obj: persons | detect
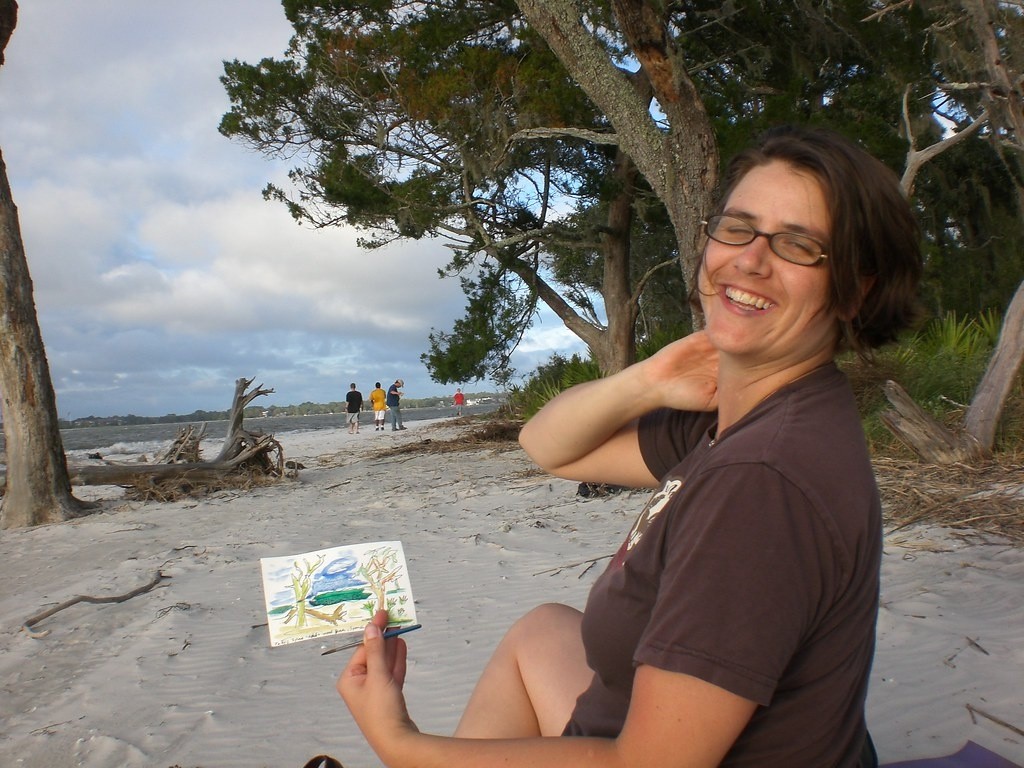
[368,382,387,431]
[345,383,364,434]
[337,129,935,768]
[387,379,408,431]
[454,387,464,417]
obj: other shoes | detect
[392,428,397,431]
[399,427,408,430]
[349,431,354,434]
[381,427,384,430]
[375,428,379,431]
[355,432,359,433]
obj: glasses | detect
[701,214,830,267]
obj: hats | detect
[397,379,404,388]
[457,388,461,391]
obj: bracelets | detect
[346,407,348,409]
